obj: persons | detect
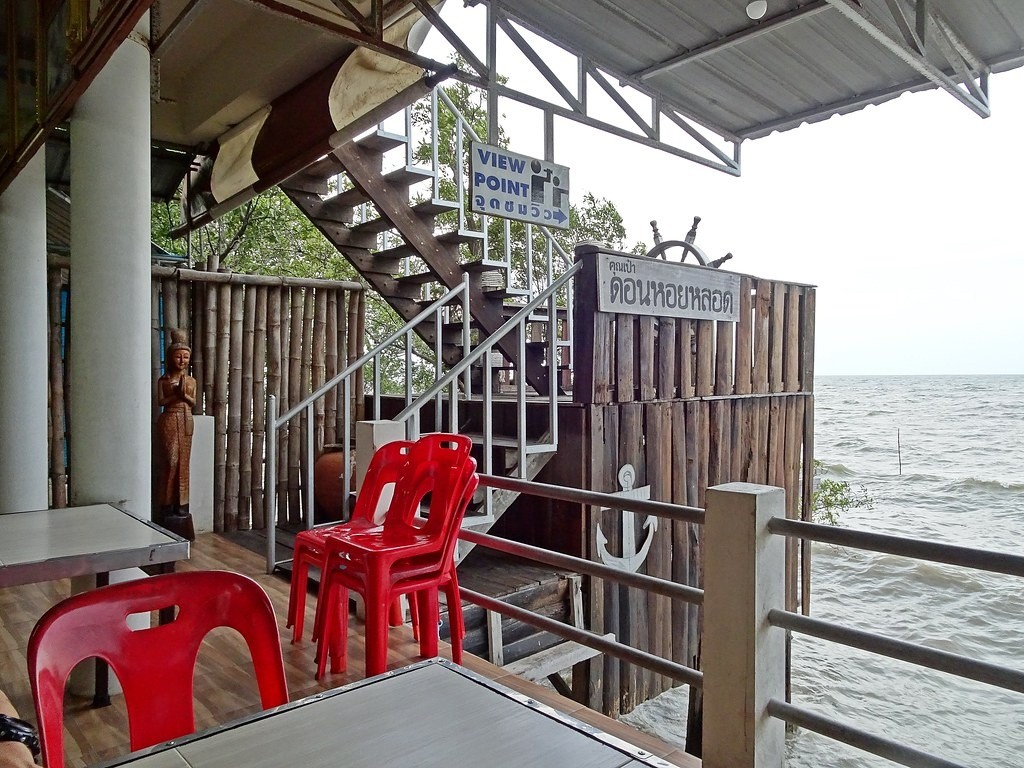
[0,689,44,768]
[158,329,197,516]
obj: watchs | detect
[0,713,41,764]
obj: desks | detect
[86,656,703,768]
[0,501,191,708]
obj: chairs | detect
[286,433,479,680]
[26,570,289,768]
